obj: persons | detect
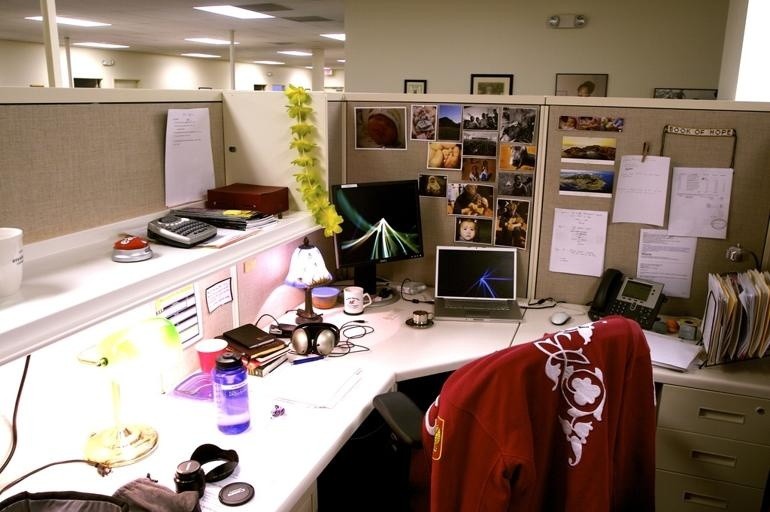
[561,115,624,131]
[577,81,595,97]
[356,103,535,247]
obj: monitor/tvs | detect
[330,178,425,309]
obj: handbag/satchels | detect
[110,478,199,512]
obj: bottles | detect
[212,350,252,435]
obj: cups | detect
[195,337,229,373]
[412,309,433,323]
[0,228,23,297]
[343,285,373,316]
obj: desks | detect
[0,87,770,511]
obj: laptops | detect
[434,244,523,323]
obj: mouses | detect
[551,311,572,325]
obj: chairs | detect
[373,315,660,512]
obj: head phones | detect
[291,322,340,357]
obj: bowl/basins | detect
[311,286,340,309]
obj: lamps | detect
[286,238,332,325]
[548,13,586,29]
[75,318,182,467]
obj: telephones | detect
[590,269,665,329]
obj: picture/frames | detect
[654,87,718,100]
[555,73,608,96]
[404,79,427,94]
[471,73,514,95]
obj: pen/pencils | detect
[292,355,323,363]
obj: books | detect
[213,322,291,378]
[170,207,279,232]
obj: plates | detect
[407,319,435,329]
